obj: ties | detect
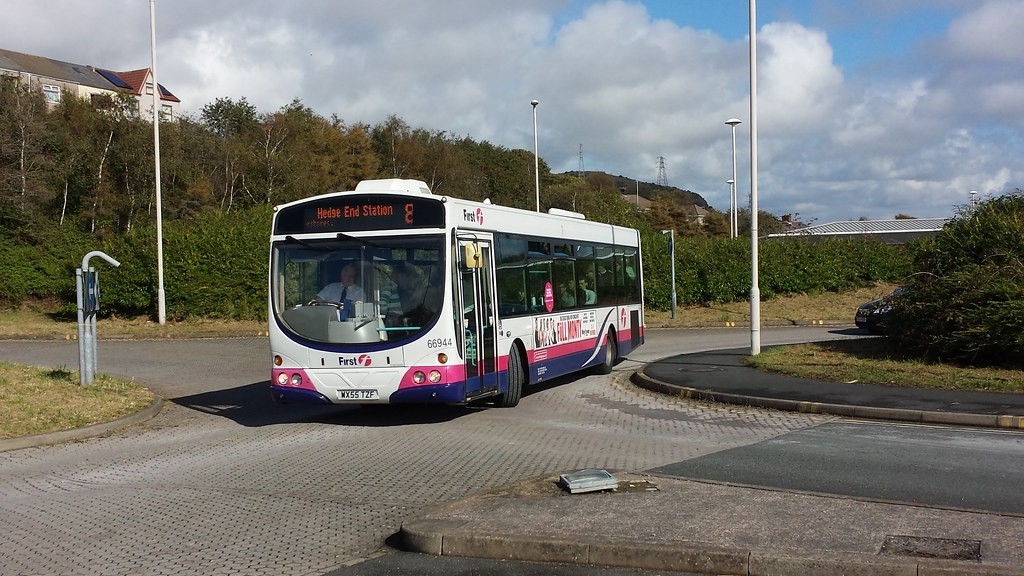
[339,286,348,306]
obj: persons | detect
[463,273,477,338]
[380,265,410,334]
[309,265,367,321]
[585,260,635,287]
[535,318,557,348]
[556,277,595,307]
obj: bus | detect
[263,178,646,415]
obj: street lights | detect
[723,118,746,237]
[531,100,543,211]
[725,179,736,240]
[661,228,681,320]
[970,190,979,217]
